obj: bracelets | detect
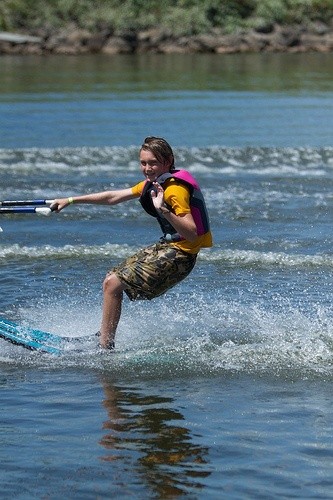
[68,197,74,205]
[158,206,171,216]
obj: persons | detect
[50,136,212,351]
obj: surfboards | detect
[1,318,98,357]
